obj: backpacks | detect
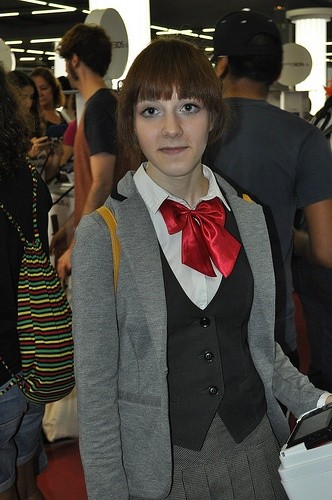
[17,241,75,405]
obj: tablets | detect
[46,124,68,141]
[287,402,332,448]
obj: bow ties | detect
[159,197,242,278]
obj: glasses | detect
[212,54,229,63]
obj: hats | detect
[209,12,283,55]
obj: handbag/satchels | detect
[42,385,82,441]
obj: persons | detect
[0,11,332,500]
[71,38,332,500]
